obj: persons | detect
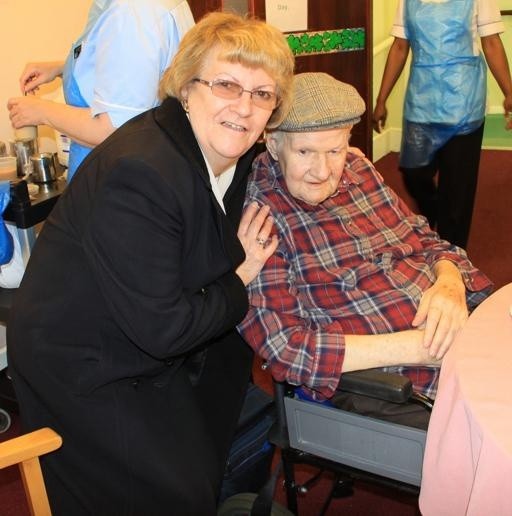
[221,70,503,430]
[367,0,512,257]
[4,9,301,516]
[6,0,186,185]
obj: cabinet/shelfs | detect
[0,177,71,410]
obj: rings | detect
[256,235,266,246]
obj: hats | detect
[265,71,366,133]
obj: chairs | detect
[0,406,64,516]
[248,355,436,515]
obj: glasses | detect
[193,77,283,110]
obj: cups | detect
[0,122,61,183]
